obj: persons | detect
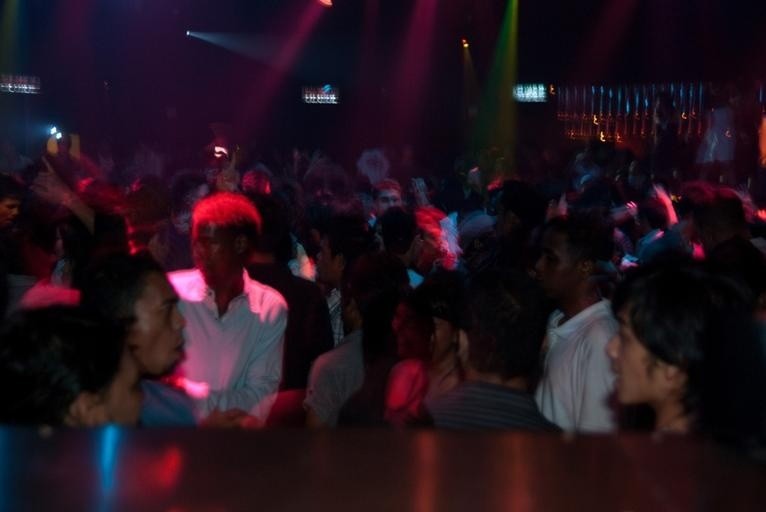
[2,85,765,434]
